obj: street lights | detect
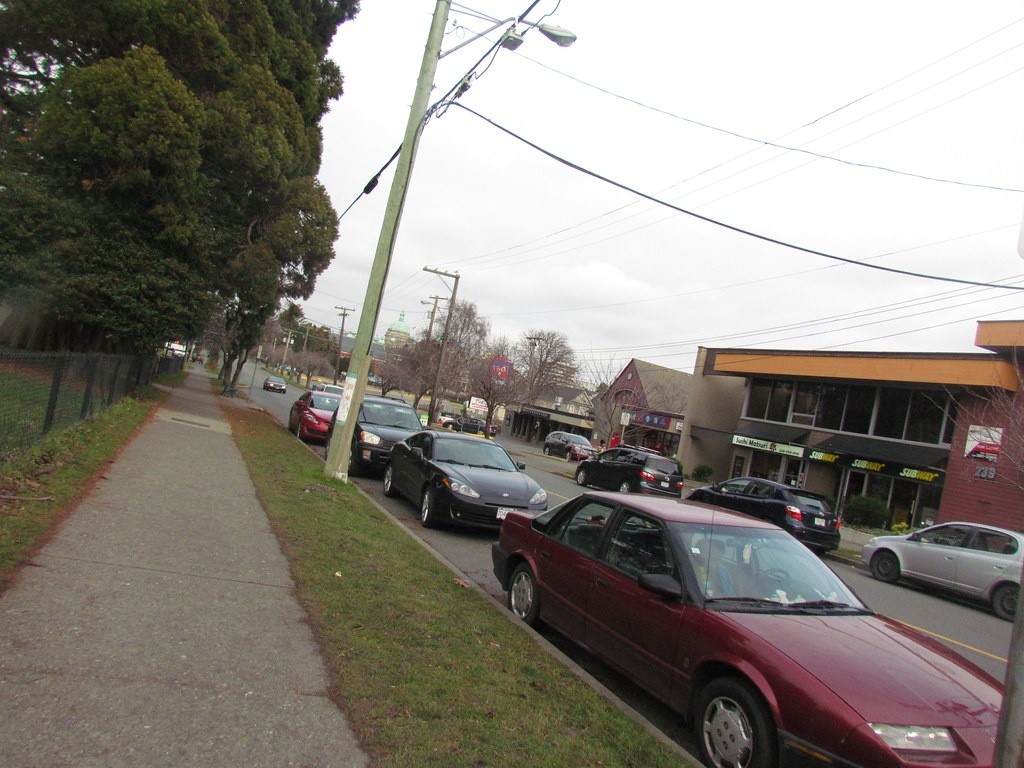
[279,337,294,367]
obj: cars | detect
[443,417,496,438]
[263,376,287,393]
[384,430,547,535]
[493,489,1006,768]
[279,365,297,371]
[861,521,1024,624]
[320,384,344,395]
[289,391,342,443]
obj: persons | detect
[597,440,606,453]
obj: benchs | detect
[567,526,662,566]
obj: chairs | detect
[696,539,732,591]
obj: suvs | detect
[685,475,842,555]
[574,444,684,500]
[542,431,593,462]
[324,394,427,477]
